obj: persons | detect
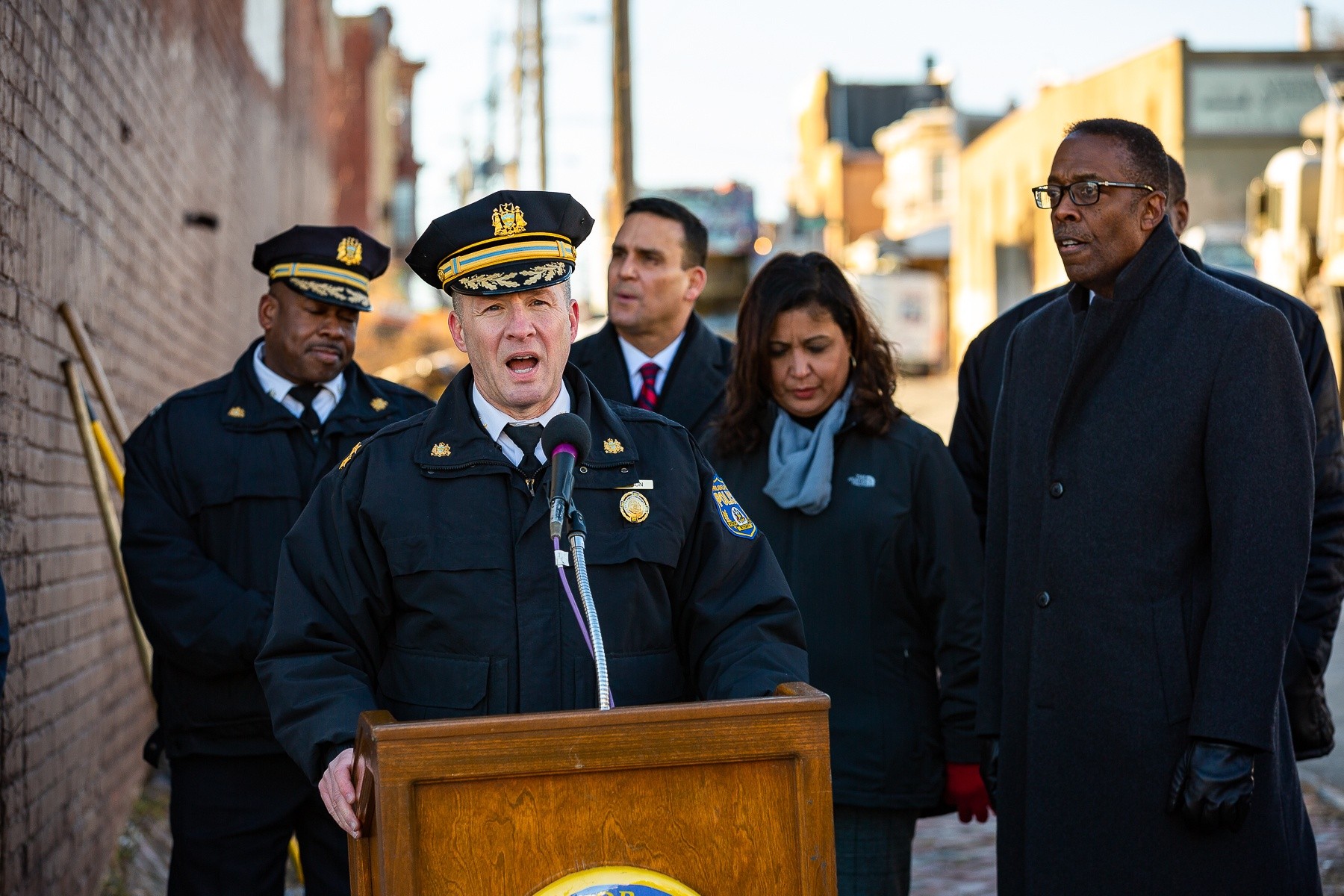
[254,190,810,841]
[1160,156,1344,763]
[119,224,437,896]
[977,118,1324,896]
[694,251,993,896]
[564,199,744,444]
[946,279,1075,798]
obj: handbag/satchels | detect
[908,809,997,896]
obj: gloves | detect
[943,760,995,824]
[1170,742,1254,823]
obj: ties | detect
[505,422,544,478]
[290,385,322,440]
[635,361,661,413]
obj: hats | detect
[404,189,601,295]
[251,223,393,312]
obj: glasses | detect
[1032,182,1155,209]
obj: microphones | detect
[542,413,591,539]
